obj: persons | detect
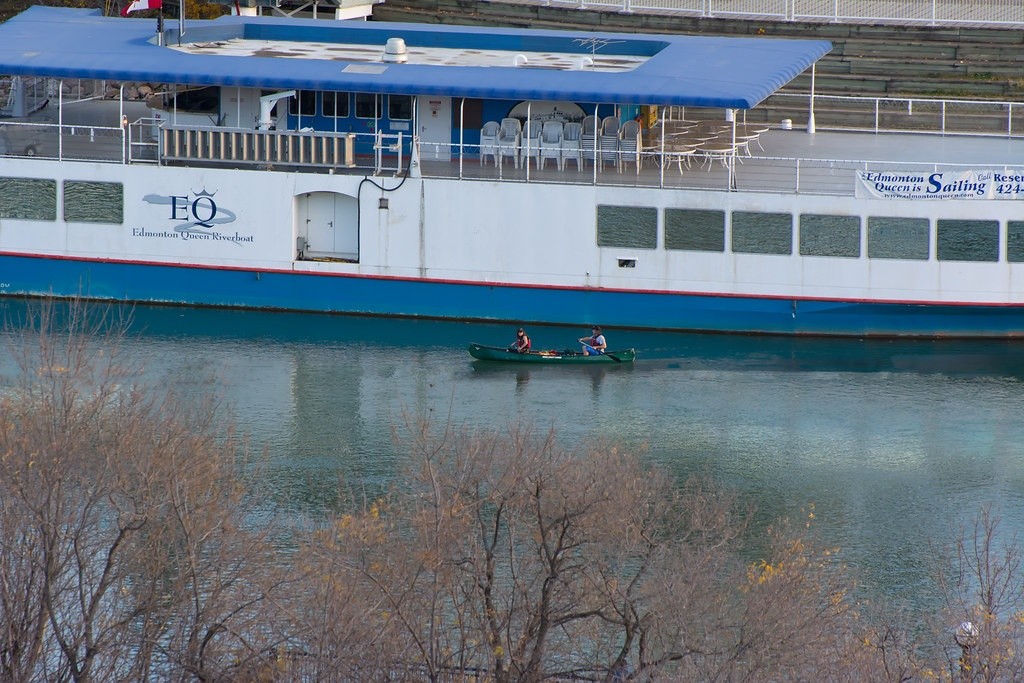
[578,326,606,356]
[506,328,531,354]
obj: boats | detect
[0,0,1022,336]
[465,339,636,364]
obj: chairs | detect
[498,118,521,169]
[521,120,542,169]
[579,115,601,174]
[562,122,581,172]
[540,121,563,171]
[619,120,642,176]
[599,116,619,175]
[479,121,499,167]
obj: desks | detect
[642,118,769,170]
[695,143,737,173]
[654,144,697,174]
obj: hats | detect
[516,327,524,331]
[592,326,601,330]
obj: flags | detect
[120,0,162,16]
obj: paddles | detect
[579,341,621,363]
